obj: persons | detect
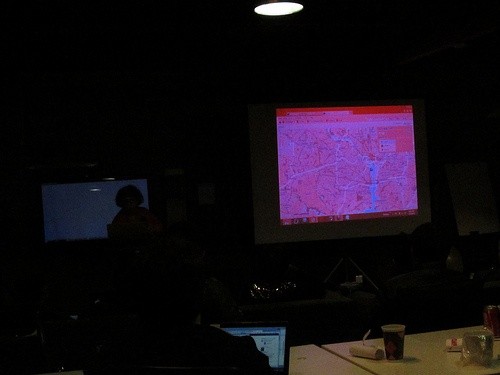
[127,269,275,375]
[106,186,158,237]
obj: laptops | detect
[220,321,289,375]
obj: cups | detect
[381,323,406,360]
[460,326,494,367]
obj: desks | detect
[289,325,500,375]
